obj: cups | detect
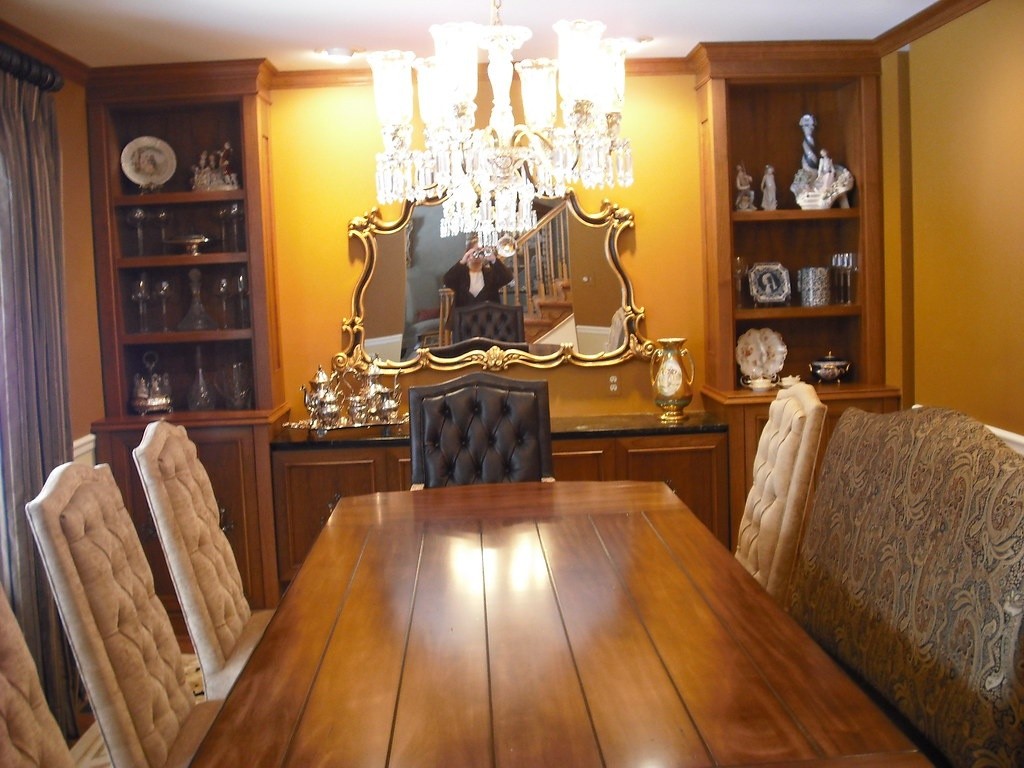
[796,266,831,307]
[215,361,253,411]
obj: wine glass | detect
[125,206,152,258]
[208,203,245,255]
[233,275,250,330]
[153,278,179,333]
[212,276,237,330]
[152,207,178,256]
[123,273,157,333]
[832,252,858,306]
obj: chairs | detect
[454,301,524,343]
[27,461,227,768]
[734,381,827,601]
[0,583,77,768]
[407,371,555,493]
[132,422,277,699]
[788,407,1024,768]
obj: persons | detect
[191,136,242,190]
[442,237,514,331]
[760,164,777,210]
[816,149,834,186]
[735,165,757,210]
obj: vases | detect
[647,339,693,422]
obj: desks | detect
[186,480,935,768]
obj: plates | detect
[120,135,177,186]
[735,328,788,377]
[745,258,793,302]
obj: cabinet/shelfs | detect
[88,409,290,626]
[269,406,731,584]
[82,60,286,418]
[699,387,899,556]
[687,40,886,397]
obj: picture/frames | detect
[748,263,792,307]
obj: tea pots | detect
[296,363,403,428]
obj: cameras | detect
[472,250,491,261]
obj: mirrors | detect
[334,155,656,372]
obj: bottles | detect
[186,346,216,410]
[174,268,220,331]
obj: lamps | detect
[368,1,635,249]
